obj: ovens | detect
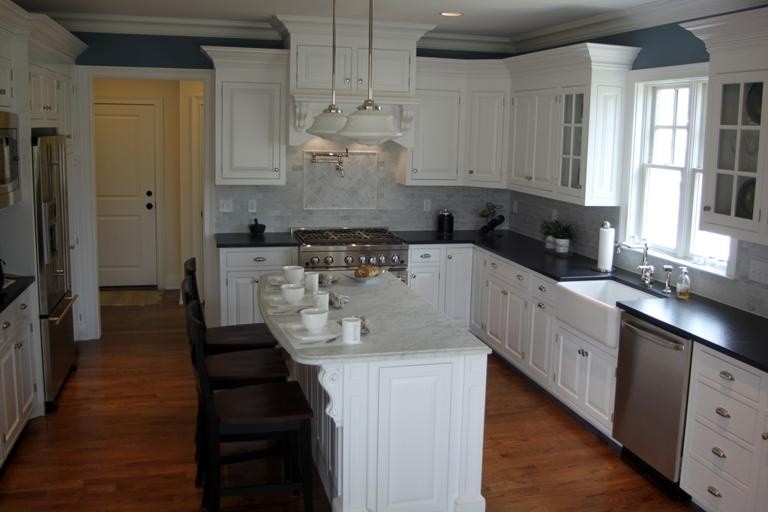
[610,310,693,485]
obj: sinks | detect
[556,279,662,348]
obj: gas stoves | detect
[290,225,408,271]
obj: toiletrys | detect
[676,266,690,300]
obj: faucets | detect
[615,242,649,280]
[663,264,673,294]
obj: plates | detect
[737,179,757,219]
[343,267,389,283]
[266,295,311,309]
[268,276,287,286]
[746,83,764,125]
[285,316,342,340]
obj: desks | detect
[253,262,490,512]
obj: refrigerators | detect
[30,133,81,413]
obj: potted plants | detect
[540,221,554,248]
[554,221,575,255]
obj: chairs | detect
[192,327,317,511]
[186,300,291,472]
[184,258,278,396]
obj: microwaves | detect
[0,113,22,214]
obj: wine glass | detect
[727,133,758,172]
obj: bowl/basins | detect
[281,265,306,283]
[299,307,330,333]
[279,282,307,305]
[248,224,266,235]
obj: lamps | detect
[304,1,348,145]
[340,1,405,149]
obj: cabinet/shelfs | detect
[551,323,623,448]
[28,63,69,117]
[0,54,18,111]
[555,42,644,208]
[508,46,555,199]
[467,245,529,393]
[529,273,554,385]
[408,241,474,331]
[680,6,768,243]
[458,59,510,192]
[396,55,461,190]
[218,248,297,335]
[197,42,289,186]
[270,13,432,109]
[675,336,767,512]
[0,286,39,465]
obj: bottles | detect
[480,215,505,234]
[675,268,691,299]
[438,207,454,239]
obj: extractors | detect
[286,95,421,151]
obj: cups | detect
[314,291,330,311]
[340,316,362,343]
[304,271,319,294]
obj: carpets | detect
[99,288,165,309]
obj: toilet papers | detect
[597,227,616,270]
